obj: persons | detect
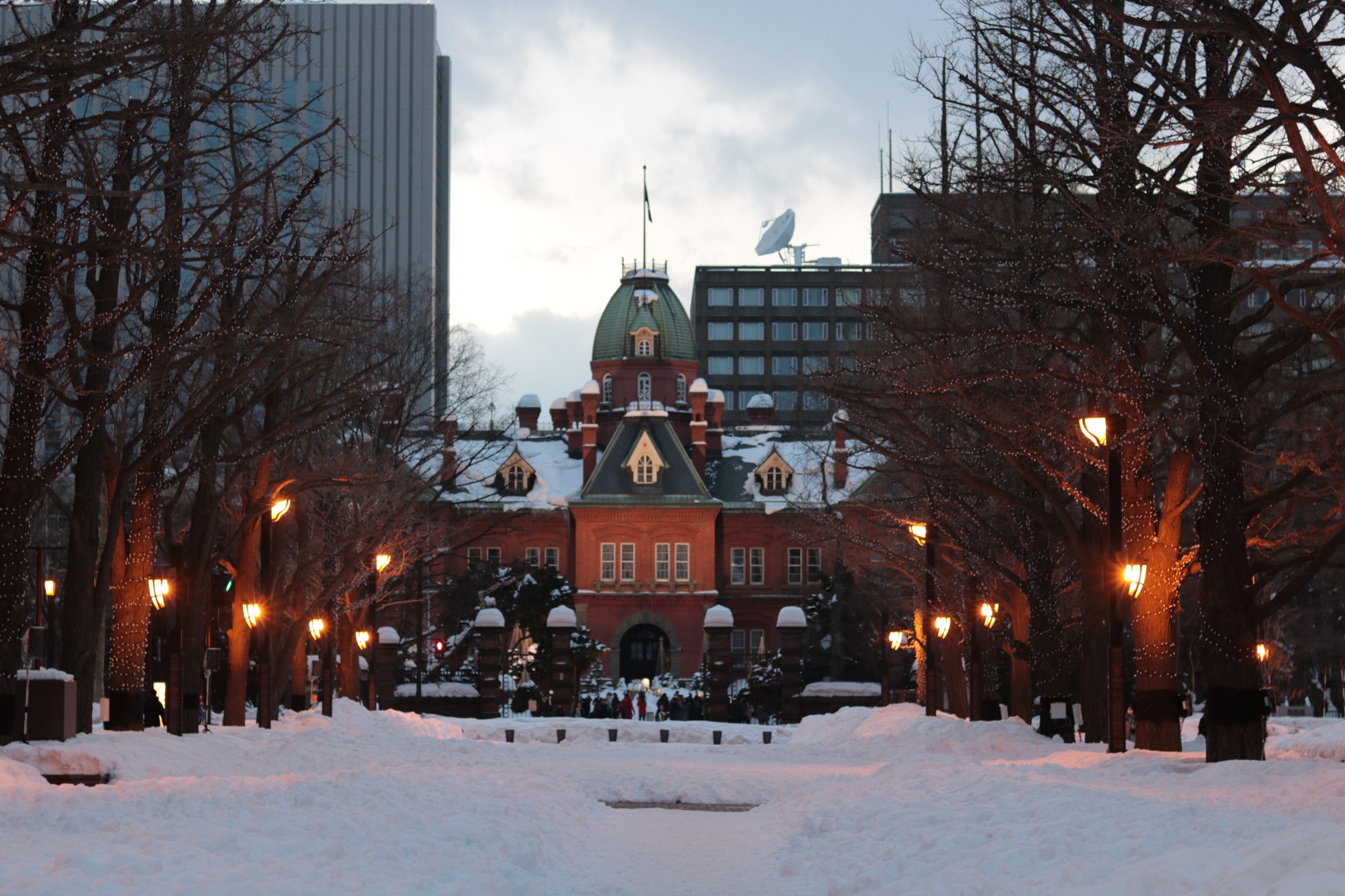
[570,690,705,722]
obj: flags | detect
[645,186,653,222]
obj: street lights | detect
[354,552,391,710]
[1076,413,1149,752]
[308,552,333,718]
[27,543,71,669]
[907,508,951,717]
[149,544,182,736]
[241,495,293,728]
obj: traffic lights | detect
[434,641,445,660]
[204,648,220,671]
[216,576,233,591]
